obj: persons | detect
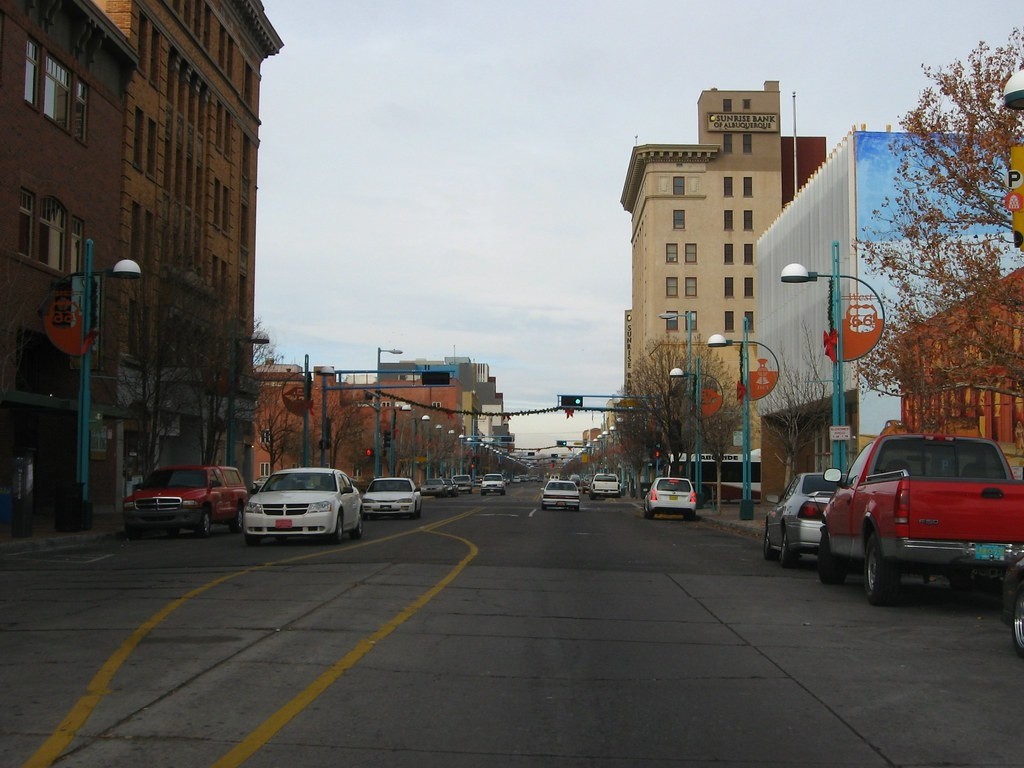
[399,483,405,491]
[320,476,330,486]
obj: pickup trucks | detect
[815,431,1024,607]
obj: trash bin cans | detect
[54,480,86,533]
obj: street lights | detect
[224,330,271,465]
[410,408,430,480]
[281,353,337,465]
[439,427,474,476]
[44,237,142,534]
[390,400,413,478]
[426,421,443,479]
[658,310,692,482]
[780,240,886,487]
[665,356,725,509]
[705,315,781,522]
[373,347,404,477]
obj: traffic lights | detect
[383,429,391,450]
[560,396,584,407]
[557,441,567,446]
[366,448,374,457]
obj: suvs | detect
[121,466,247,539]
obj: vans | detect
[480,474,507,495]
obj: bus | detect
[664,448,763,505]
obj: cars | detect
[361,478,423,519]
[540,479,581,511]
[503,474,543,486]
[419,474,482,498]
[241,467,363,543]
[642,477,698,522]
[570,473,622,501]
[762,473,850,569]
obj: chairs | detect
[884,459,911,476]
[962,463,982,478]
